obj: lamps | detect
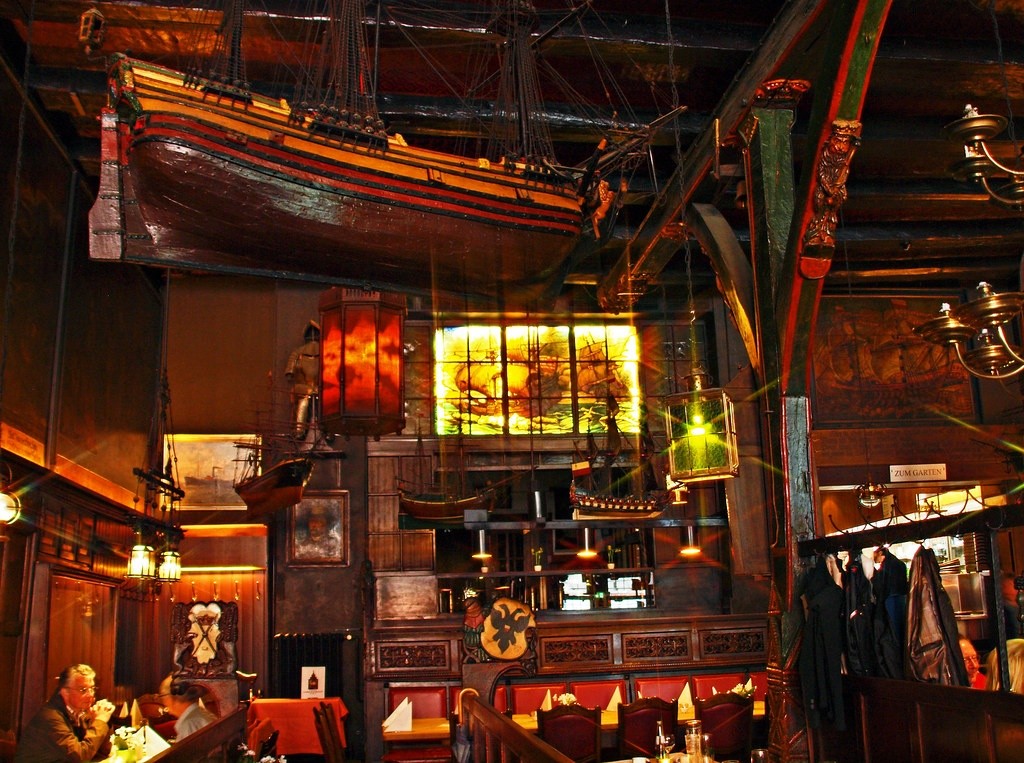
[664,0,1024,510]
[0,283,409,582]
[536,490,542,517]
[471,526,701,558]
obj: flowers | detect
[530,547,544,565]
[727,683,758,698]
[607,545,621,563]
[552,693,580,706]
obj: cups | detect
[751,749,769,763]
[685,734,714,763]
[632,757,646,763]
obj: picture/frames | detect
[284,489,350,570]
[552,530,596,556]
[161,433,262,511]
[809,285,986,431]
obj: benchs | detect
[384,672,768,763]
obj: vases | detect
[607,563,615,569]
[534,565,542,572]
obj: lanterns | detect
[665,388,740,482]
[318,288,406,440]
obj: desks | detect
[108,705,176,729]
[380,701,766,763]
[246,697,349,755]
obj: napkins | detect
[130,698,143,728]
[382,696,409,727]
[541,689,553,710]
[678,682,693,703]
[606,686,622,711]
[385,701,413,732]
[119,701,129,718]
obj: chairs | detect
[694,693,754,763]
[618,695,679,759]
[537,705,601,763]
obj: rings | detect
[92,709,97,712]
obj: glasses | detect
[64,685,98,695]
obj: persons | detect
[958,633,1024,695]
[15,664,115,763]
[160,675,217,744]
